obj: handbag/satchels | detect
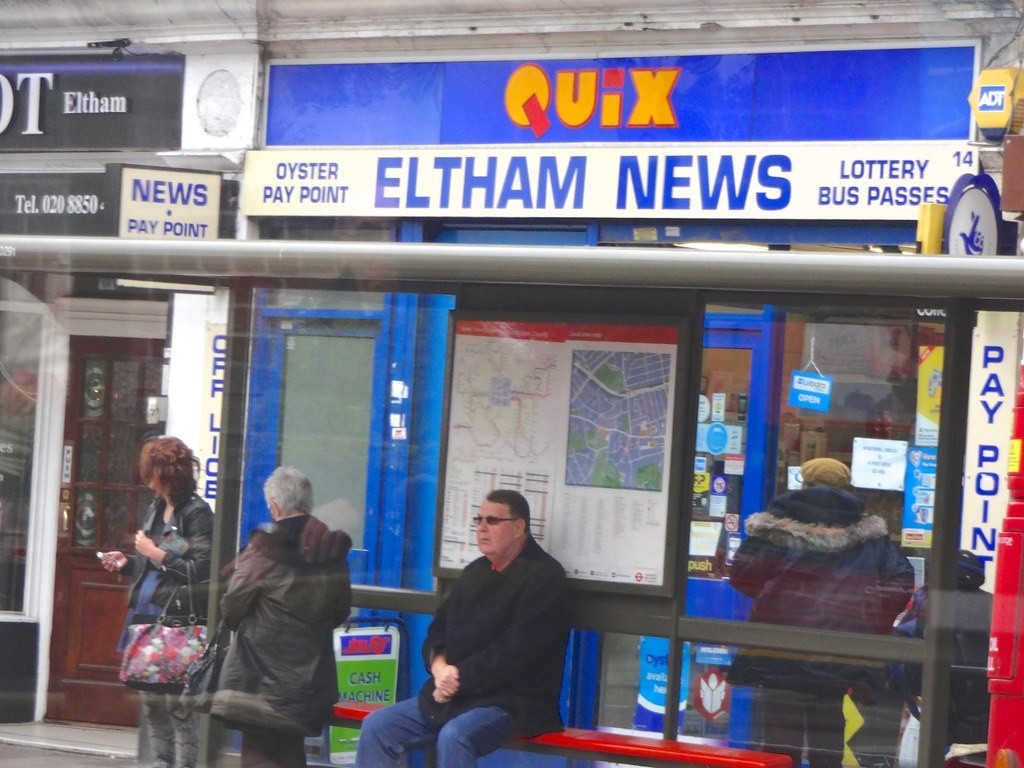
[177,619,233,714]
[119,559,207,684]
[419,678,468,730]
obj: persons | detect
[725,457,915,768]
[99,435,215,768]
[354,488,573,768]
[208,465,353,768]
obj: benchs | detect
[332,700,794,768]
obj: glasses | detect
[473,514,517,526]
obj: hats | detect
[800,458,851,487]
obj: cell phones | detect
[97,552,121,567]
